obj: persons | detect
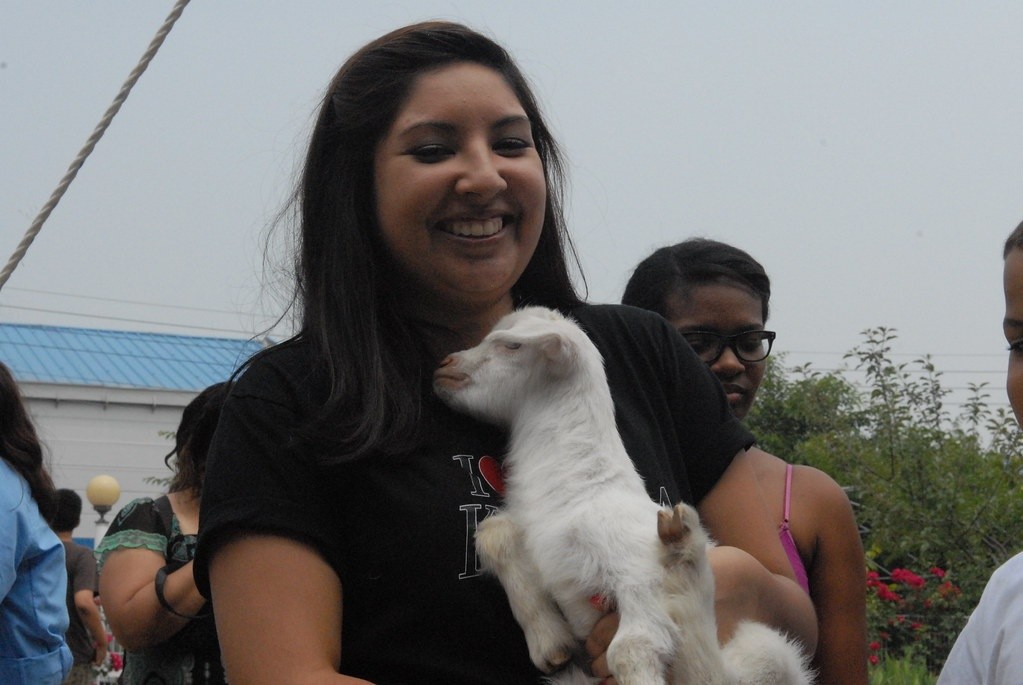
[0,363,75,685]
[620,239,871,685]
[192,20,820,685]
[53,489,108,685]
[90,382,226,685]
[937,222,1023,685]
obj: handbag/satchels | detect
[154,532,202,623]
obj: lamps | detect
[85,474,122,519]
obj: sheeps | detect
[432,307,821,685]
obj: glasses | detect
[679,331,775,363]
